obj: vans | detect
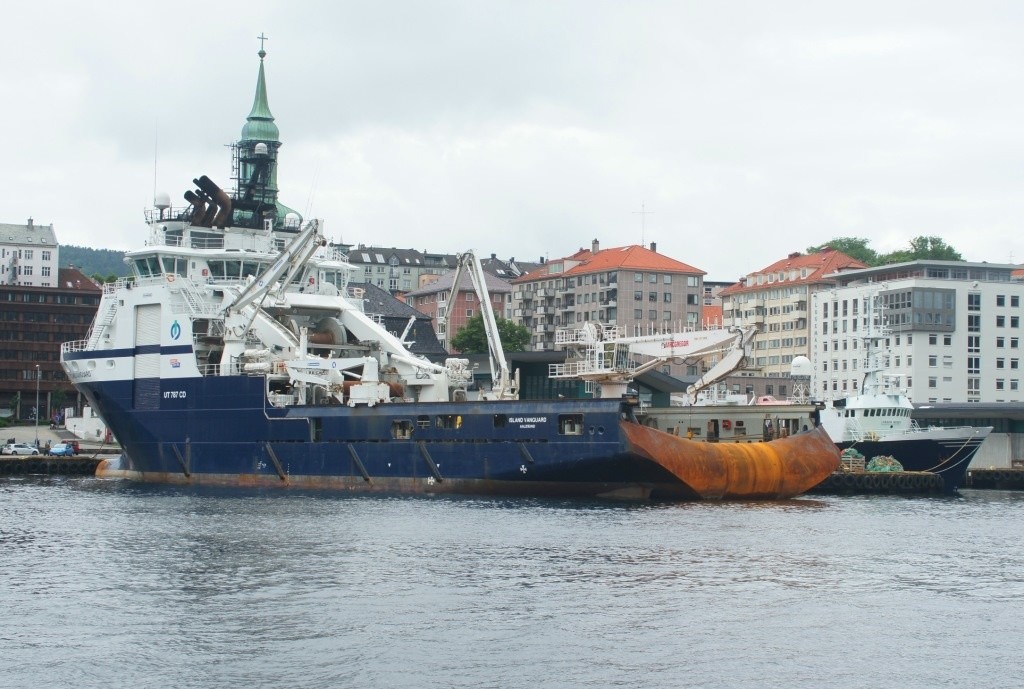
[60,439,79,455]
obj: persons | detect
[44,440,52,456]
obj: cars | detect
[23,442,46,453]
[49,444,74,457]
[2,444,39,455]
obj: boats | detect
[60,142,842,501]
[806,283,995,496]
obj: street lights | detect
[35,365,40,447]
